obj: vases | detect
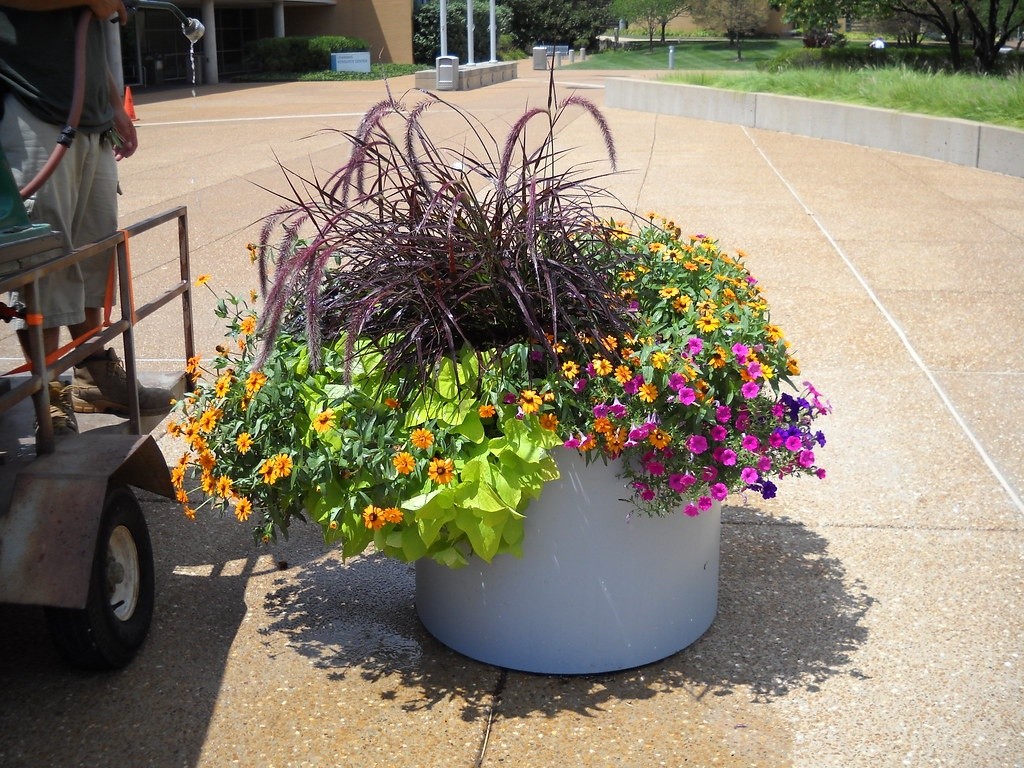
[416,444,722,676]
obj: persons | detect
[869,35,885,49]
[0,0,175,441]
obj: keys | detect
[102,126,126,149]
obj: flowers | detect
[157,40,829,567]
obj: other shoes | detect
[70,347,176,416]
[32,381,81,443]
